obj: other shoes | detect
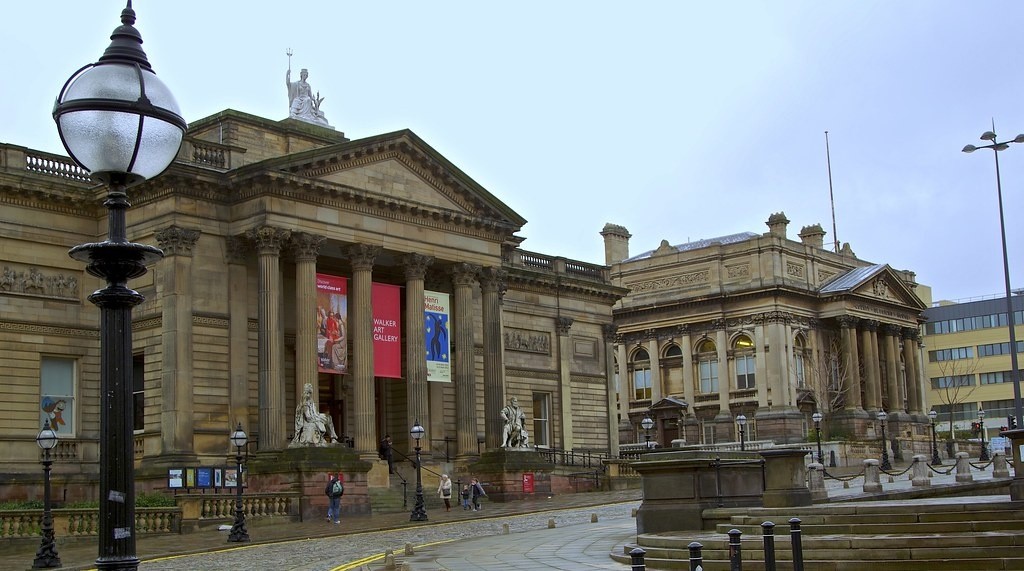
[390,472,394,474]
[477,504,481,510]
[448,507,450,511]
[444,511,448,512]
[469,506,471,509]
[334,520,340,525]
[473,509,477,511]
[463,508,466,510]
[327,517,330,523]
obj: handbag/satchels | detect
[443,489,450,496]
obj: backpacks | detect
[333,480,342,493]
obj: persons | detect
[500,397,530,449]
[286,68,318,111]
[461,485,472,511]
[436,474,452,512]
[317,308,345,368]
[469,478,489,512]
[379,434,395,475]
[325,473,344,526]
[302,383,338,444]
[505,331,549,352]
[0,267,77,298]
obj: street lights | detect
[52,0,190,571]
[410,418,430,522]
[736,411,747,451]
[641,412,655,450]
[960,117,1024,429]
[811,411,823,464]
[31,417,64,571]
[876,407,893,471]
[227,421,252,543]
[927,406,943,465]
[977,407,990,462]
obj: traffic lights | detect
[1001,426,1007,431]
[1009,416,1016,429]
[976,423,980,430]
